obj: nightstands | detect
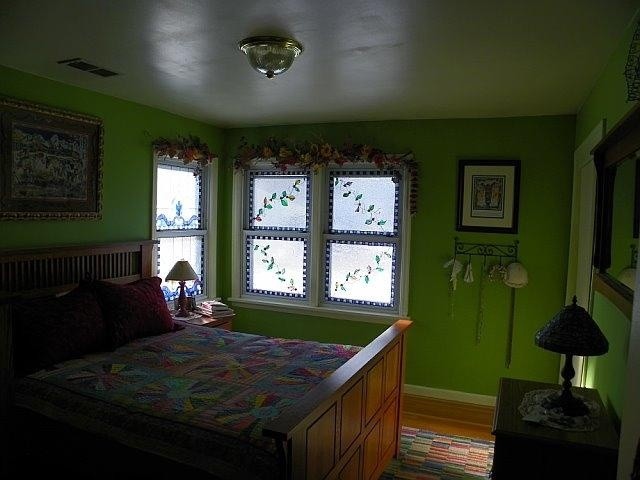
[171,309,235,333]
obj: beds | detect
[2,240,414,480]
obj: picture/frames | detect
[1,96,104,223]
[455,159,522,234]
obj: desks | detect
[491,378,620,480]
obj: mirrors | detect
[590,103,639,322]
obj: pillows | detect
[6,277,187,381]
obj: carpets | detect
[379,423,495,480]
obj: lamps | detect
[166,260,199,318]
[237,37,303,79]
[534,295,609,419]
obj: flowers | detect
[147,129,218,169]
[230,133,407,173]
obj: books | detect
[194,301,235,319]
